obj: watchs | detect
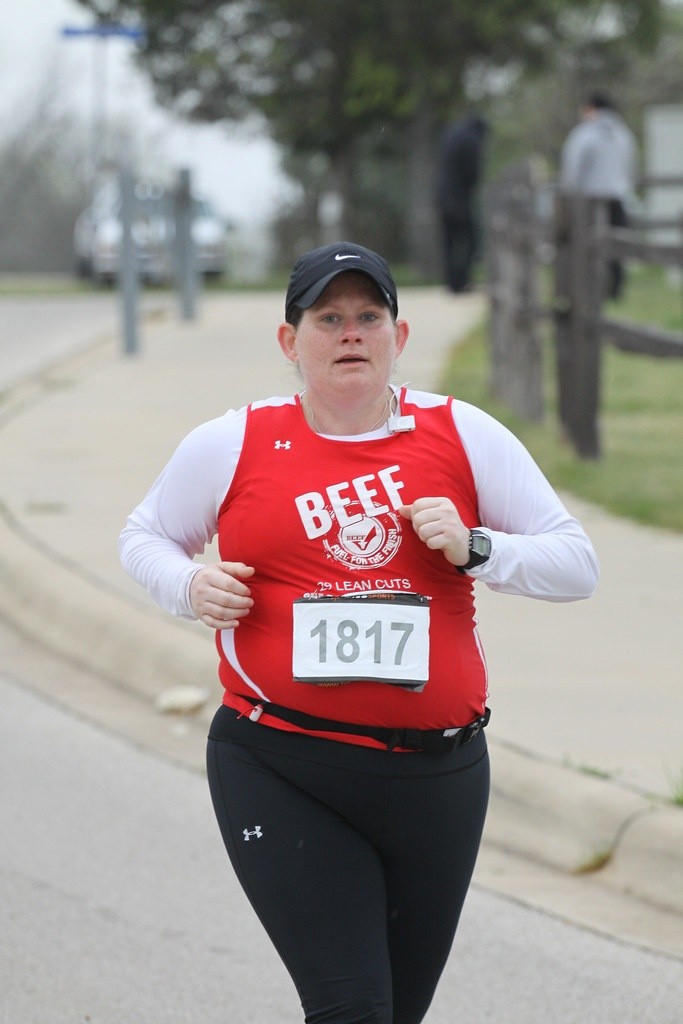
[455,530,491,574]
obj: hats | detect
[284,241,397,323]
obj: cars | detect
[74,179,228,289]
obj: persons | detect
[118,242,600,1024]
[554,92,639,295]
[433,115,490,294]
